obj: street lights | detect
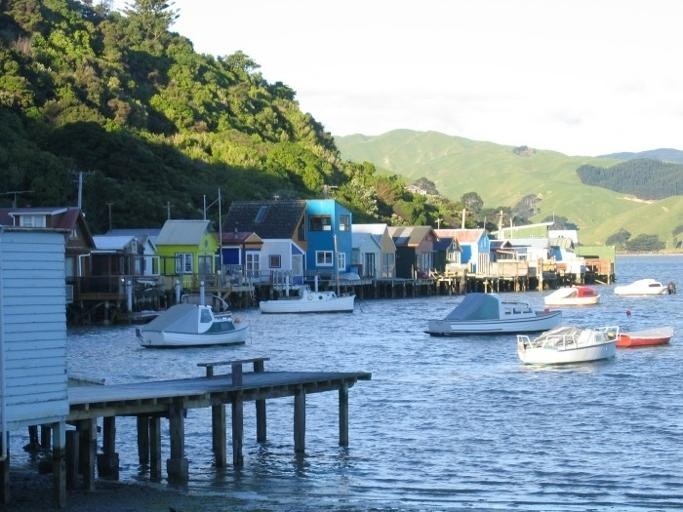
[203,195,221,221]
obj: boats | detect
[136,302,251,346]
[515,324,617,363]
[618,325,676,346]
[260,290,354,312]
[428,292,565,336]
[615,279,676,296]
[547,285,598,305]
